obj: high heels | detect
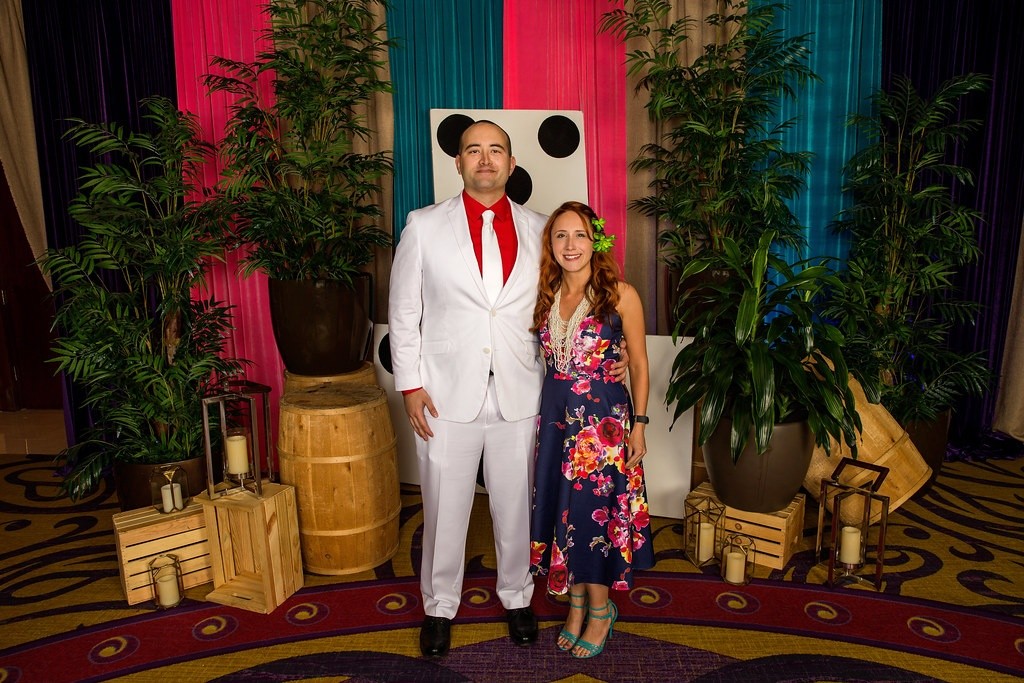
[572,598,617,658]
[558,594,589,652]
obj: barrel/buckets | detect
[280,361,378,403]
[790,346,933,526]
[277,386,402,575]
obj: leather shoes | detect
[507,605,538,646]
[419,615,452,657]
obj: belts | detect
[490,370,494,375]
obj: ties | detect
[482,210,503,305]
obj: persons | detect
[388,119,629,661]
[530,201,656,658]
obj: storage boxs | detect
[110,501,213,605]
[683,481,806,569]
[193,476,306,615]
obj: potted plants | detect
[209,0,403,375]
[828,73,996,487]
[601,0,860,517]
[32,96,238,512]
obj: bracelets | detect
[632,415,649,424]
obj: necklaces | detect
[548,285,595,374]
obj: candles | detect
[726,552,747,583]
[225,434,250,475]
[158,576,180,608]
[695,523,716,560]
[161,483,181,511]
[841,527,861,565]
